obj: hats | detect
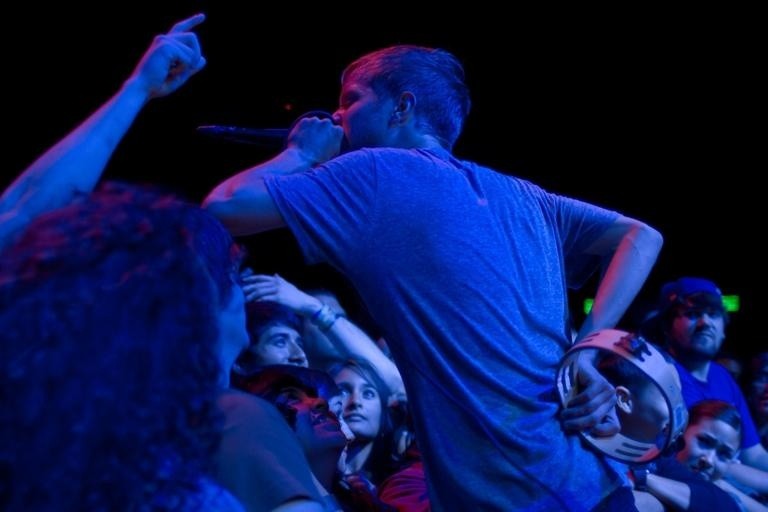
[660,277,724,310]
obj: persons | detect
[2,186,767,511]
[200,33,674,510]
[1,8,215,235]
